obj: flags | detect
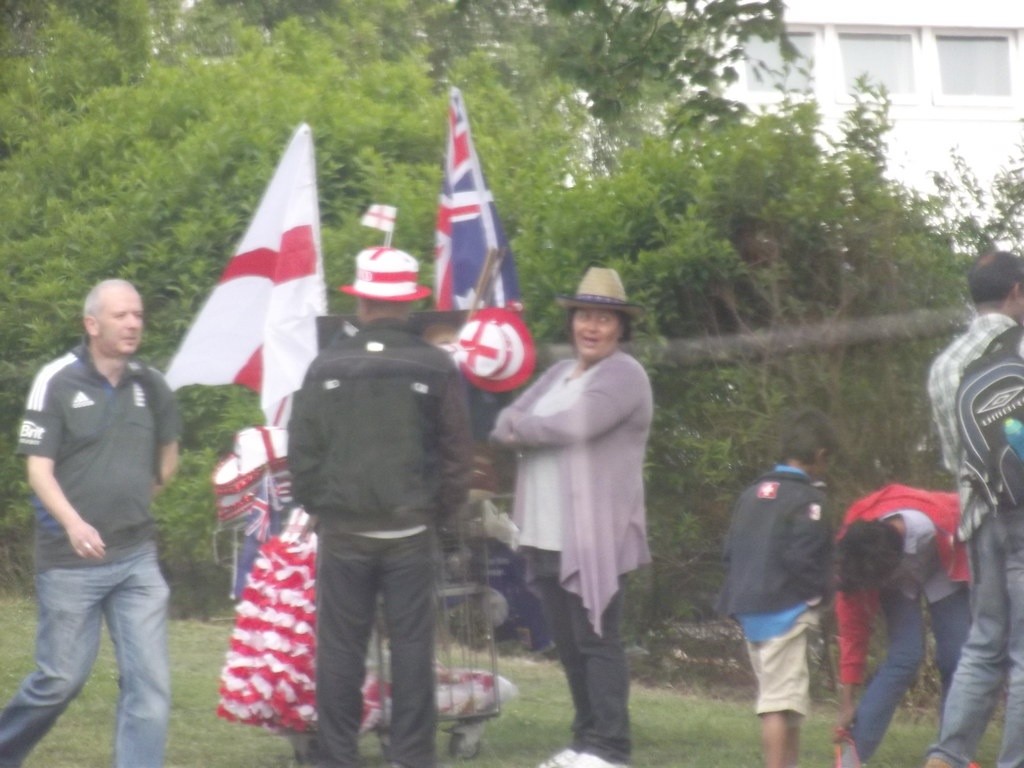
[361,205,397,232]
[431,86,522,316]
[163,123,328,429]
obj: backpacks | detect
[956,325,1024,513]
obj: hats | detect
[556,265,646,321]
[340,248,432,302]
[457,309,537,392]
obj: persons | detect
[286,244,472,764]
[486,267,656,768]
[714,411,842,768]
[926,251,1024,767]
[0,276,181,768]
[831,483,984,767]
[458,454,563,665]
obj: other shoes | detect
[538,748,629,768]
[925,756,981,768]
[836,736,861,768]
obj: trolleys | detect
[221,468,504,768]
